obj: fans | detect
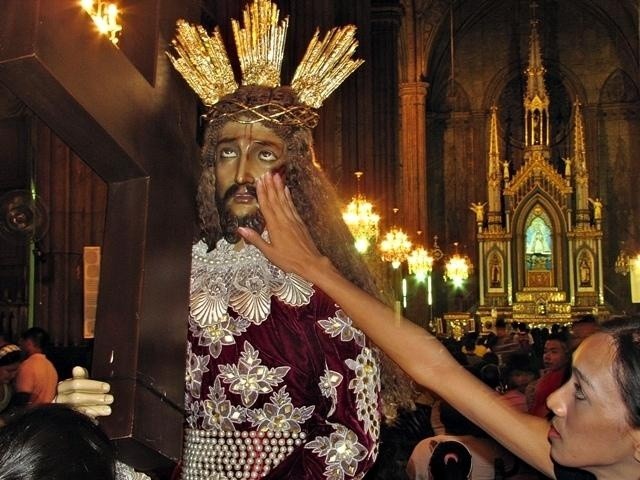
[0,187,50,262]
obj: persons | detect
[452,316,601,420]
[50,0,381,480]
[0,327,119,480]
[404,399,501,479]
[237,172,639,479]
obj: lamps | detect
[379,205,411,268]
[407,228,436,286]
[343,169,381,255]
[442,242,474,283]
[78,1,125,48]
